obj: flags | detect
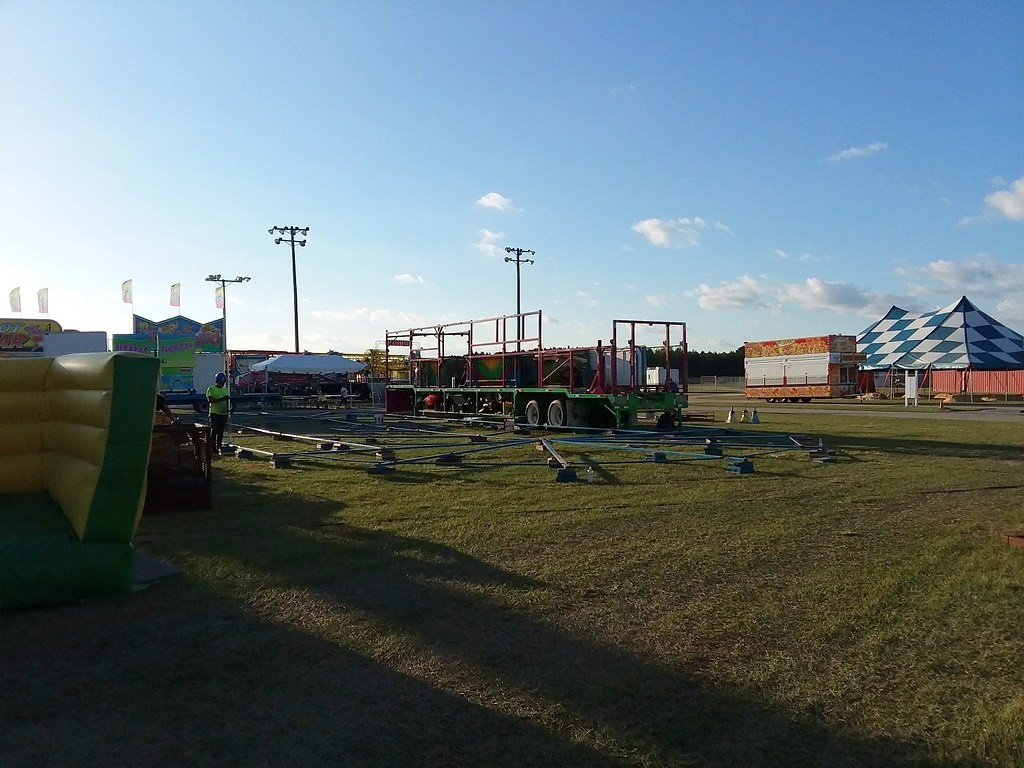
[9,279,224,313]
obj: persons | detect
[669,379,679,393]
[156,373,349,455]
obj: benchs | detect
[283,399,362,408]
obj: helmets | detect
[215,373,227,382]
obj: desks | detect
[311,394,360,408]
[282,396,310,408]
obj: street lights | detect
[268,225,310,356]
[206,274,251,353]
[504,246,535,352]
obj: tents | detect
[853,295,1024,404]
[250,354,369,408]
